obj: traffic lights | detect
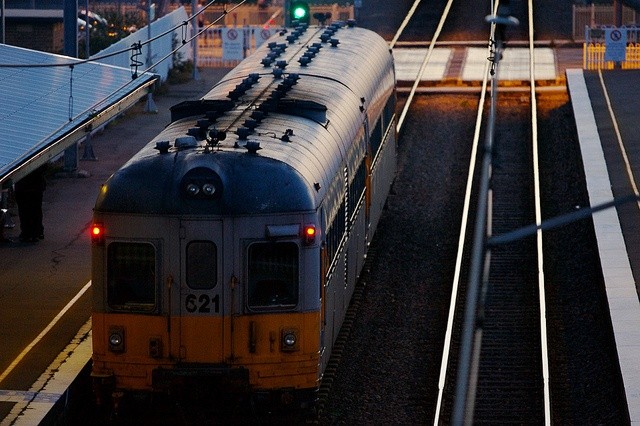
[283,2,312,25]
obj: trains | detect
[86,18,406,413]
